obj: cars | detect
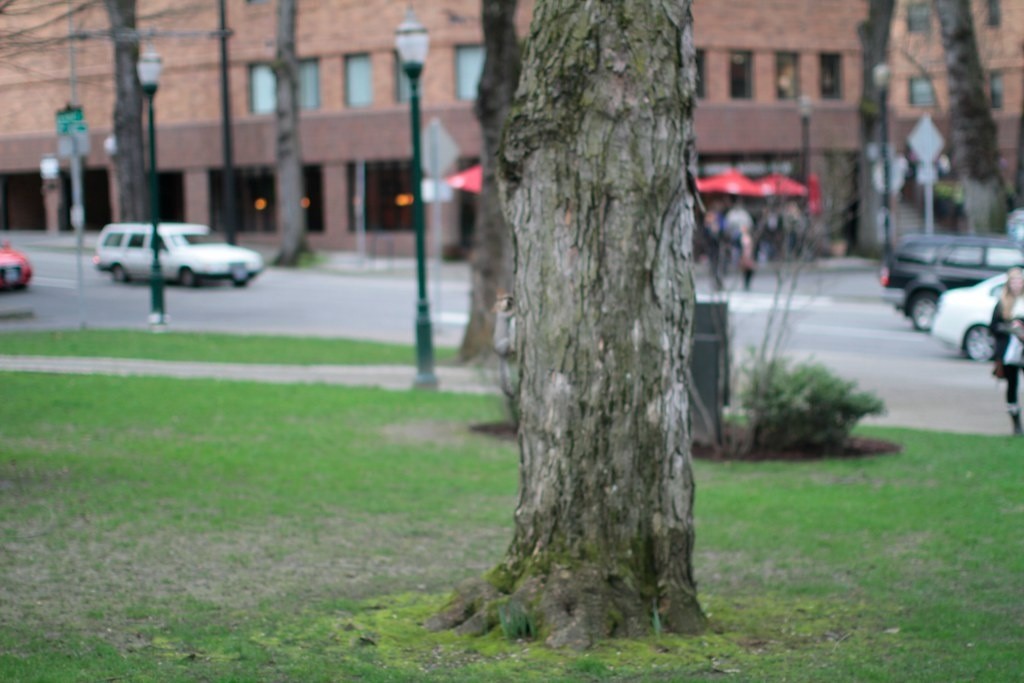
[932,272,1010,363]
[0,240,32,291]
[95,223,264,288]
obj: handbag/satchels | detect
[1002,334,1024,366]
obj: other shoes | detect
[1012,414,1022,435]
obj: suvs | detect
[874,236,1024,333]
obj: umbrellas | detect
[446,165,482,194]
[751,173,809,196]
[695,170,761,196]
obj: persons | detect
[705,200,760,288]
[987,265,1024,434]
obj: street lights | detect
[395,4,440,392]
[104,133,124,223]
[799,92,810,203]
[136,40,164,325]
[872,61,891,257]
